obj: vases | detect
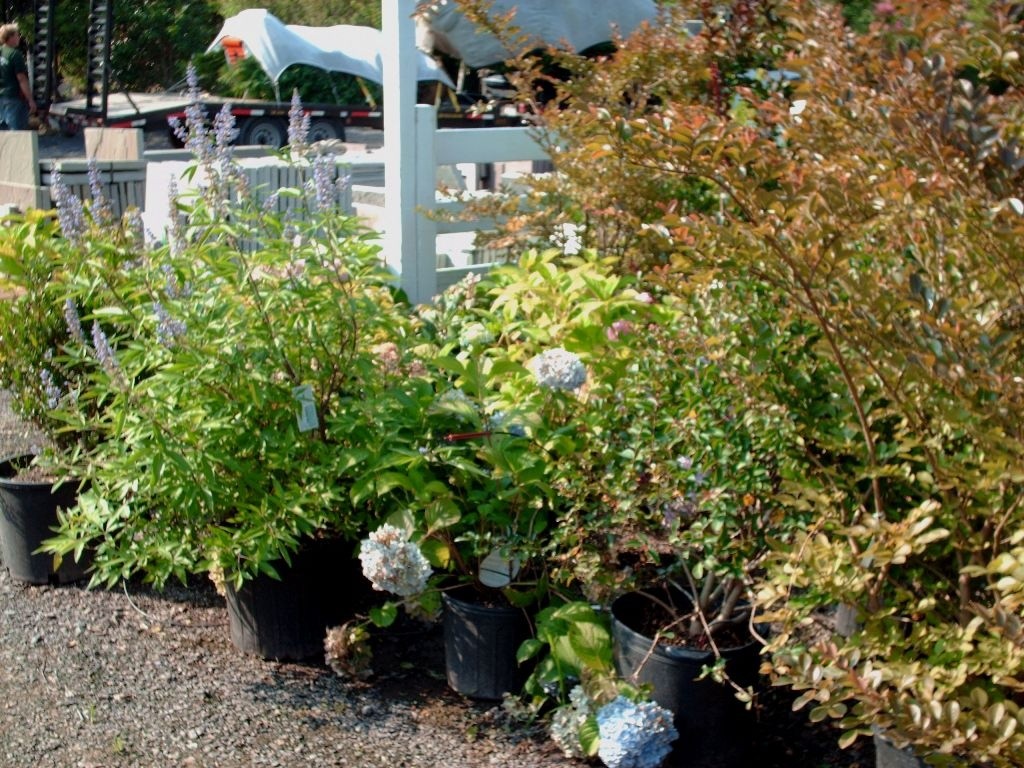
[618,580,769,741]
[0,453,103,584]
[871,693,983,768]
[439,556,577,698]
[222,509,389,665]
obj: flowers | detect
[0,58,1024,768]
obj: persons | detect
[0,23,36,131]
